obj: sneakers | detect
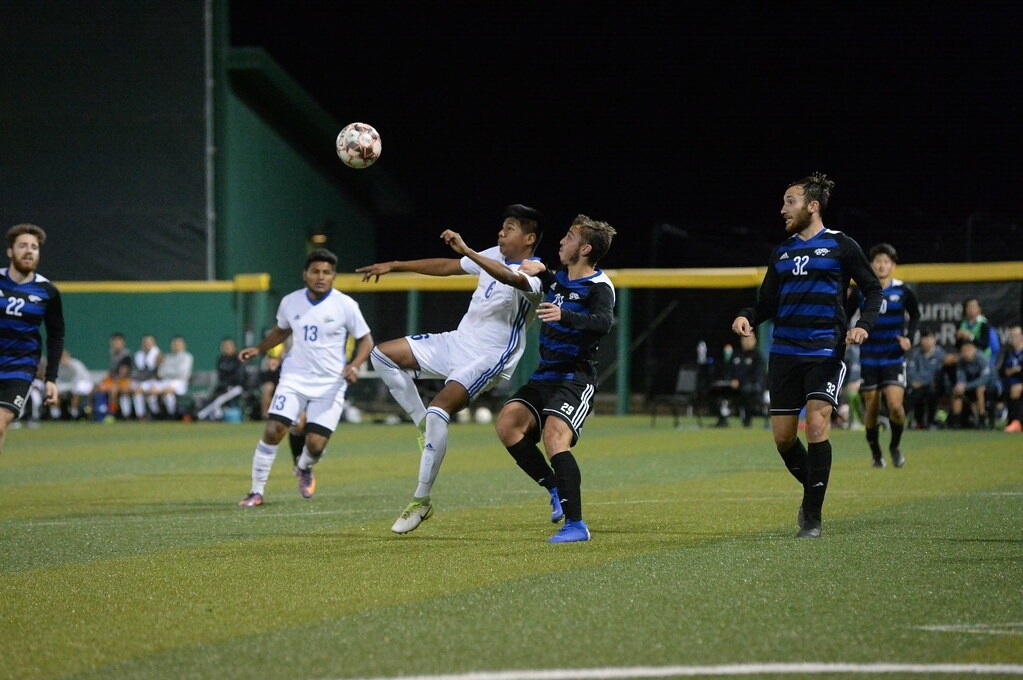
[391,501,433,534]
[548,518,590,543]
[417,414,427,452]
[238,493,263,507]
[550,487,563,523]
[295,456,316,497]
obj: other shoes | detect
[797,527,822,539]
[874,457,887,468]
[798,505,805,527]
[890,443,905,468]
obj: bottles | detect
[697,341,707,364]
[724,345,733,362]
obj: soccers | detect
[335,122,382,169]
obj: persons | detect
[49,352,95,422]
[96,333,132,422]
[355,205,544,533]
[732,172,885,538]
[133,335,164,422]
[495,214,616,544]
[239,249,374,507]
[714,330,765,427]
[907,296,1023,430]
[195,338,247,421]
[260,342,283,420]
[1,225,65,451]
[157,336,193,420]
[847,243,922,469]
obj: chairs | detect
[651,362,704,429]
